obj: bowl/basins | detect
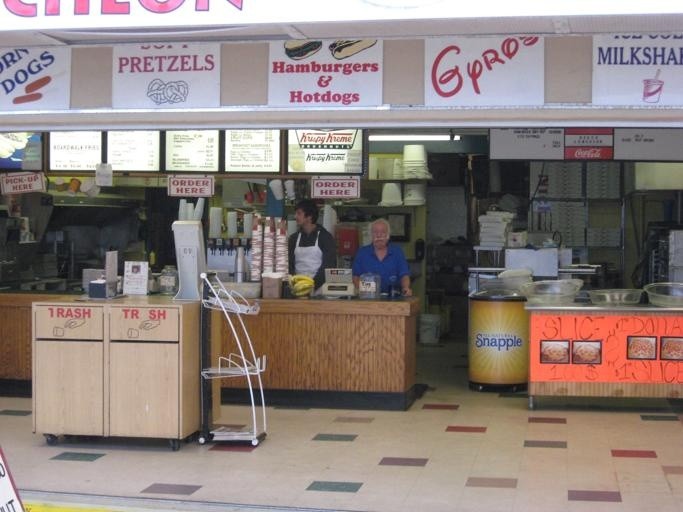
[520,279,681,311]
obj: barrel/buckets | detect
[418,313,441,344]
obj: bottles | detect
[159,265,179,296]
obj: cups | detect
[247,214,291,281]
[55,177,100,198]
[269,180,295,202]
[392,158,403,180]
[177,195,250,241]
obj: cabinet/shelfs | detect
[32,295,199,451]
[201,269,266,449]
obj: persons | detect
[351,220,412,299]
[288,202,337,292]
